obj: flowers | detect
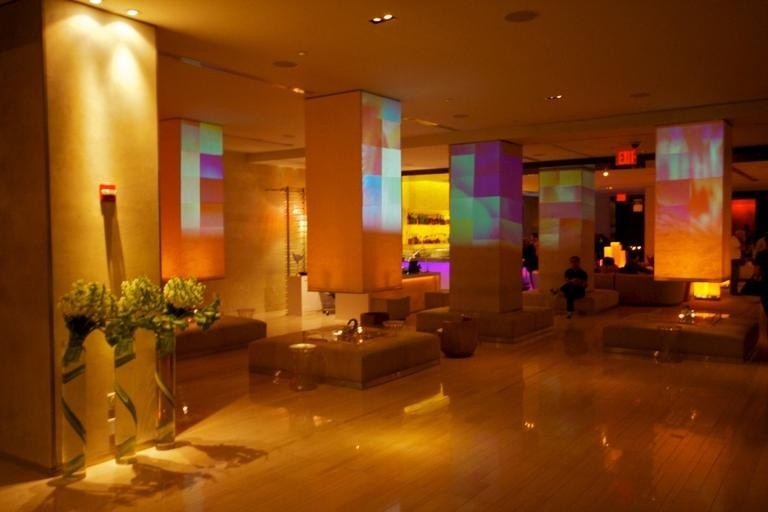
[57,275,222,367]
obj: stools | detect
[369,292,410,320]
[425,288,449,306]
[654,325,682,362]
[288,343,317,392]
[442,318,479,359]
[237,308,256,319]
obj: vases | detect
[113,338,136,464]
[60,349,86,480]
[155,329,176,451]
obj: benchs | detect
[594,272,692,305]
[416,306,554,343]
[523,289,619,314]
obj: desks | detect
[176,315,267,361]
[603,313,760,364]
[248,324,441,390]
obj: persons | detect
[522,230,540,290]
[550,256,588,319]
[593,251,654,274]
[730,229,768,316]
